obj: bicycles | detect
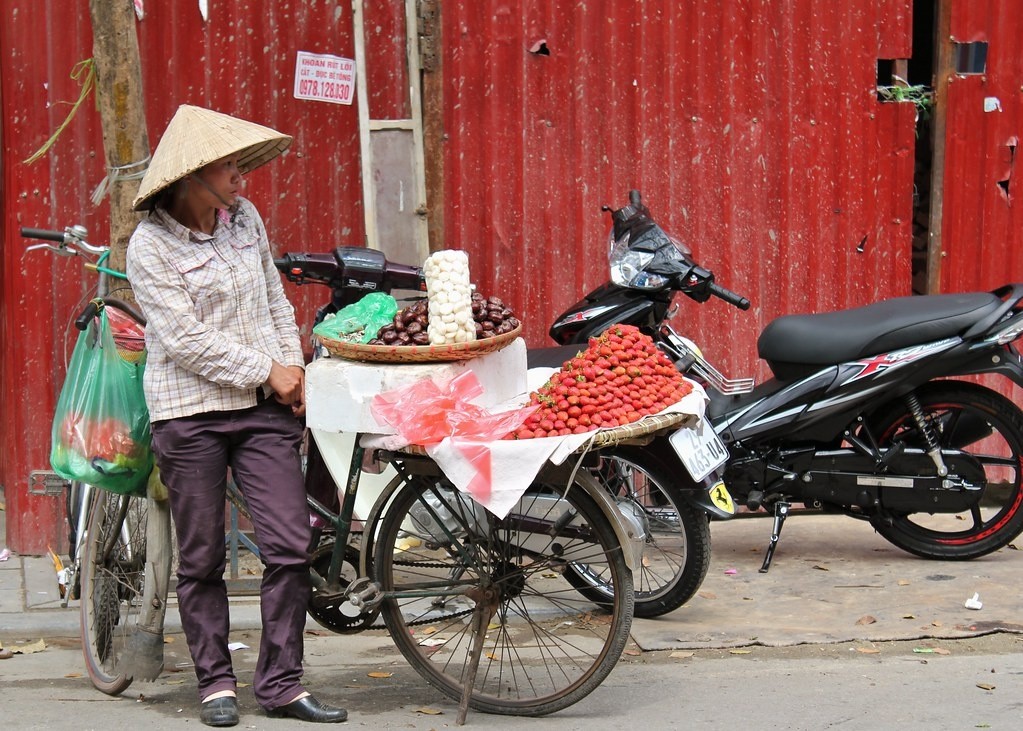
[11,222,642,727]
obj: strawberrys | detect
[498,323,694,439]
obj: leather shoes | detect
[266,694,348,722]
[199,696,239,726]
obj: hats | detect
[133,105,294,211]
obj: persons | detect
[125,104,348,726]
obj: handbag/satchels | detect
[50,297,154,497]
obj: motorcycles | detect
[548,190,1023,574]
[270,248,744,615]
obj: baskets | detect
[311,305,522,364]
[534,378,704,449]
